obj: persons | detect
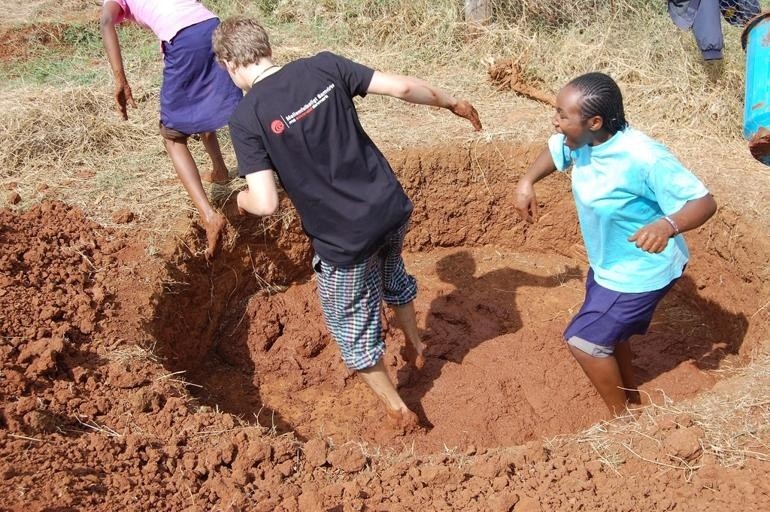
[209,16,482,432]
[99,0,236,261]
[511,70,720,424]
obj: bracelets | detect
[659,216,679,238]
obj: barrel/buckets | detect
[742,11,766,163]
